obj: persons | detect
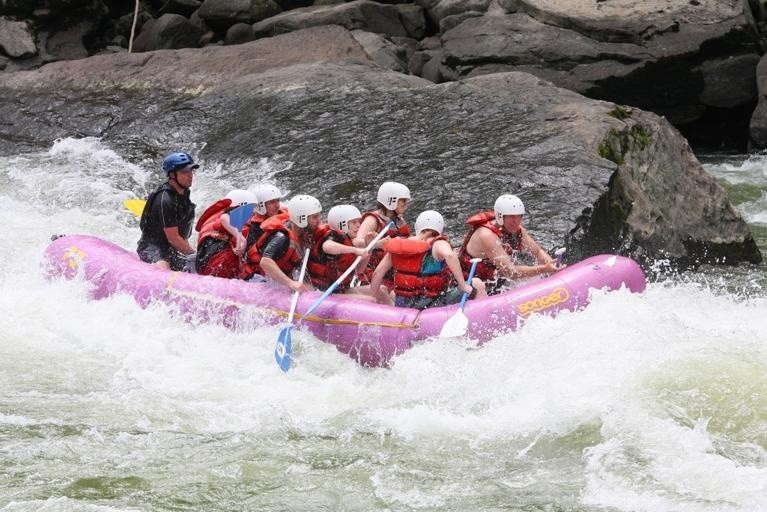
[353,182,413,291]
[247,183,289,278]
[195,188,257,279]
[301,203,394,307]
[259,193,323,293]
[370,210,488,309]
[137,152,201,271]
[448,194,568,297]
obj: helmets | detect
[376,181,411,211]
[248,184,282,216]
[328,204,363,235]
[494,195,525,226]
[224,189,258,211]
[415,210,444,236]
[162,153,200,177]
[288,195,323,228]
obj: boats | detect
[39,235,646,369]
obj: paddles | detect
[439,257,482,337]
[304,221,397,320]
[275,247,312,373]
[123,198,147,217]
[230,203,257,272]
